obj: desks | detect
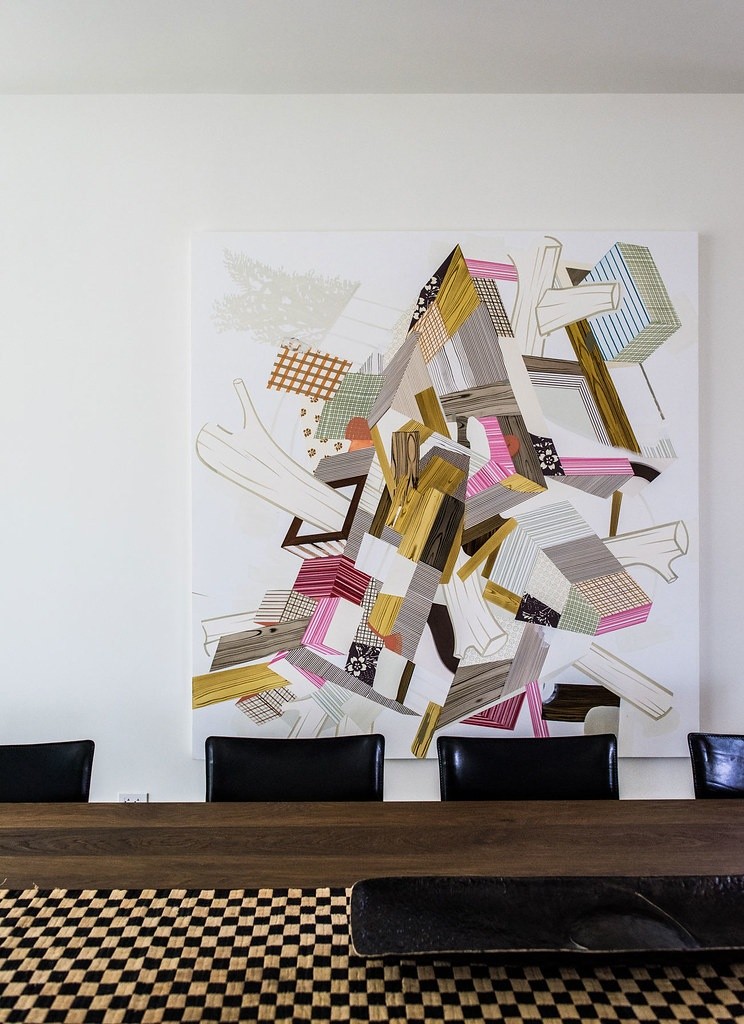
[0,799,744,1024]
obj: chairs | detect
[205,733,385,800]
[688,733,744,800]
[437,734,619,802]
[0,740,95,802]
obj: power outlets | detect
[119,793,147,803]
